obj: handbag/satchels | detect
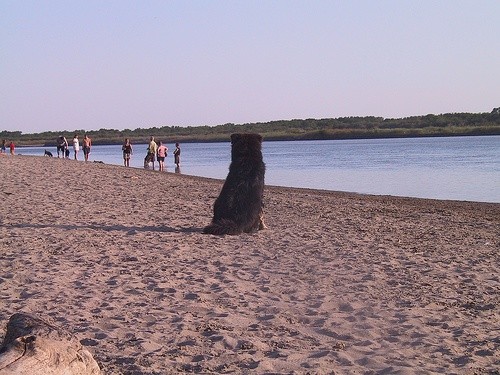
[165,152,167,157]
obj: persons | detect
[1,138,6,153]
[9,141,15,155]
[173,143,180,168]
[63,136,69,158]
[56,135,65,158]
[82,133,91,162]
[144,135,158,170]
[72,134,79,160]
[121,138,133,167]
[157,141,168,171]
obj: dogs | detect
[65,148,70,157]
[204,133,268,235]
[44,150,53,157]
